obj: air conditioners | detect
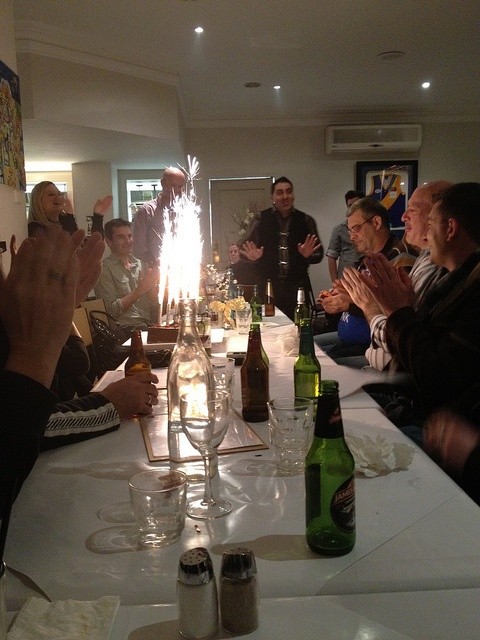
[324,123,423,155]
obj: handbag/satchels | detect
[89,310,126,359]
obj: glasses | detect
[348,216,374,234]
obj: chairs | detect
[307,274,326,320]
[80,297,130,368]
[68,307,101,376]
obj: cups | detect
[129,469,187,547]
[267,397,314,475]
[196,317,212,357]
[180,391,235,520]
[236,309,252,336]
[211,357,235,407]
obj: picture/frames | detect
[355,159,418,236]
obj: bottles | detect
[240,330,271,422]
[177,546,219,633]
[304,379,356,556]
[221,546,258,629]
[168,299,217,461]
[294,318,321,410]
[251,285,263,322]
[171,298,176,309]
[178,288,184,318]
[124,330,153,417]
[294,287,309,336]
[265,278,276,316]
[186,290,190,298]
[250,324,269,365]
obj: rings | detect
[147,394,152,405]
[49,270,63,281]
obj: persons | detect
[95,217,160,333]
[338,179,461,371]
[27,181,115,237]
[0,227,107,527]
[45,372,158,451]
[59,321,92,394]
[325,190,365,283]
[356,184,479,504]
[316,198,420,370]
[133,167,186,264]
[238,176,325,321]
[228,243,240,265]
